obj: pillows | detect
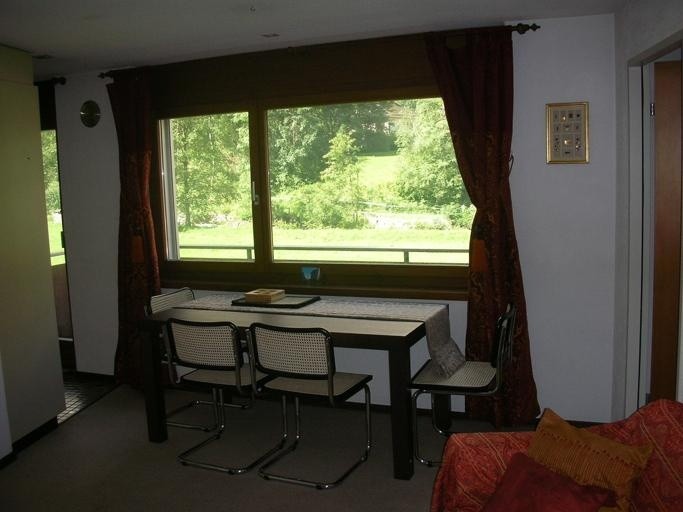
[528,406,654,511]
[482,452,615,511]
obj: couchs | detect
[426,397,682,511]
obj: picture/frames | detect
[543,101,592,166]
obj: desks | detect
[170,292,452,482]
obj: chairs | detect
[406,298,520,469]
[240,319,378,491]
[145,283,255,432]
[166,313,292,477]
